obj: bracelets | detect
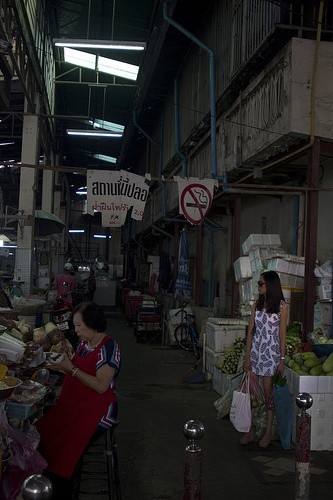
[71,367,79,376]
[280,356,286,360]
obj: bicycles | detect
[173,301,200,360]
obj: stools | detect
[72,420,122,500]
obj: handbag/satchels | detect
[214,371,266,433]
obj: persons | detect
[53,263,74,301]
[33,302,120,490]
[239,270,288,448]
[1,264,14,283]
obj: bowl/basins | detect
[42,352,65,366]
[0,376,23,401]
[312,343,333,358]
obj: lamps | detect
[53,0,147,194]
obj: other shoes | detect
[239,434,255,445]
[257,437,273,448]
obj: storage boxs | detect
[166,308,194,346]
[6,400,36,418]
[202,234,333,450]
[122,287,160,332]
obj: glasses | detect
[258,280,266,287]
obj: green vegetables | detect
[285,323,308,349]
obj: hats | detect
[64,262,73,271]
